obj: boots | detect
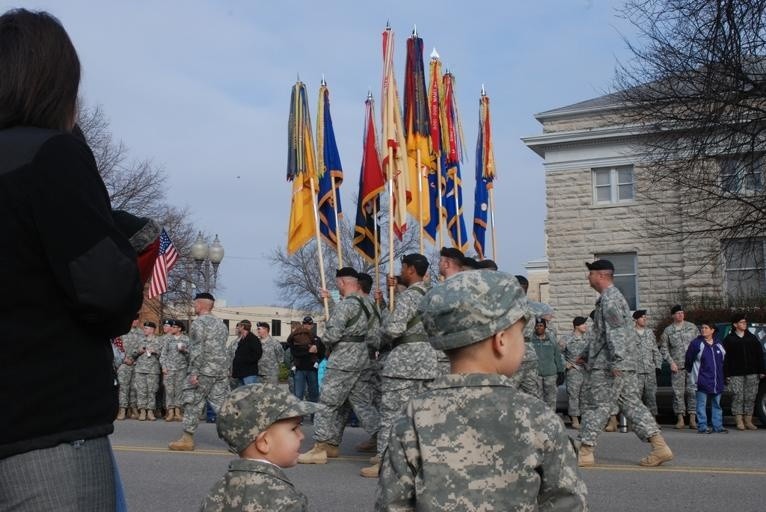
[734,414,758,430]
[639,416,674,467]
[116,408,184,422]
[606,416,617,431]
[356,432,379,478]
[168,431,195,450]
[571,416,579,427]
[296,441,339,463]
[675,414,699,428]
[576,445,596,467]
[199,407,207,420]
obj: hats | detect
[403,254,428,265]
[257,322,269,328]
[418,270,551,350]
[303,317,314,323]
[573,310,595,325]
[336,267,373,281]
[195,293,214,301]
[671,305,682,315]
[133,314,185,329]
[441,249,497,269]
[215,382,325,454]
[585,260,613,270]
[632,310,647,319]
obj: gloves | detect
[556,373,563,387]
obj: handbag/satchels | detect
[342,334,365,343]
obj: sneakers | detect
[206,419,215,422]
[698,426,727,433]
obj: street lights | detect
[190,229,225,296]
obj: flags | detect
[286,28,497,264]
[148,229,179,299]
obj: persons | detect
[661,305,766,433]
[298,248,562,476]
[559,308,663,432]
[373,269,590,512]
[0,6,144,512]
[107,248,285,451]
[288,316,326,426]
[201,382,327,512]
[575,259,674,466]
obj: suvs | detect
[553,321,766,423]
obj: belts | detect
[391,333,431,349]
